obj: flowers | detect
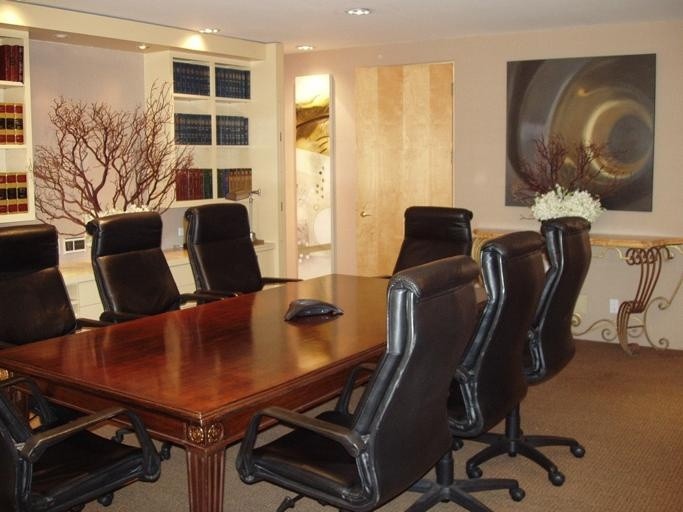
[527,184,607,226]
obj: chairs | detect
[453,217,593,486]
[364,232,549,511]
[229,259,479,512]
[378,205,475,279]
[90,210,225,460]
[1,221,118,435]
[1,378,164,512]
[180,203,305,298]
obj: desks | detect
[466,228,683,358]
[0,272,396,512]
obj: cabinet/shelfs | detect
[144,50,265,207]
[0,26,38,226]
